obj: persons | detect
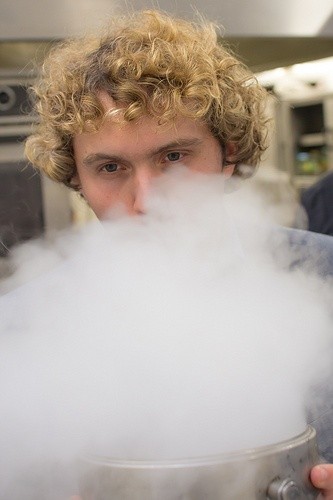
[21,7,333,500]
